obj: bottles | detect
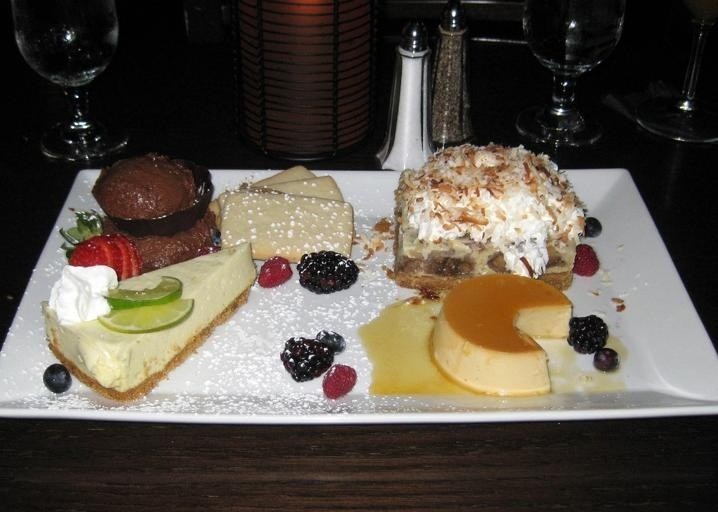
[370,16,440,170]
[430,1,478,151]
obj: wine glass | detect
[6,0,132,162]
[627,0,717,142]
[507,0,623,150]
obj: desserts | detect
[39,142,619,402]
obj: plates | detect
[0,161,717,425]
